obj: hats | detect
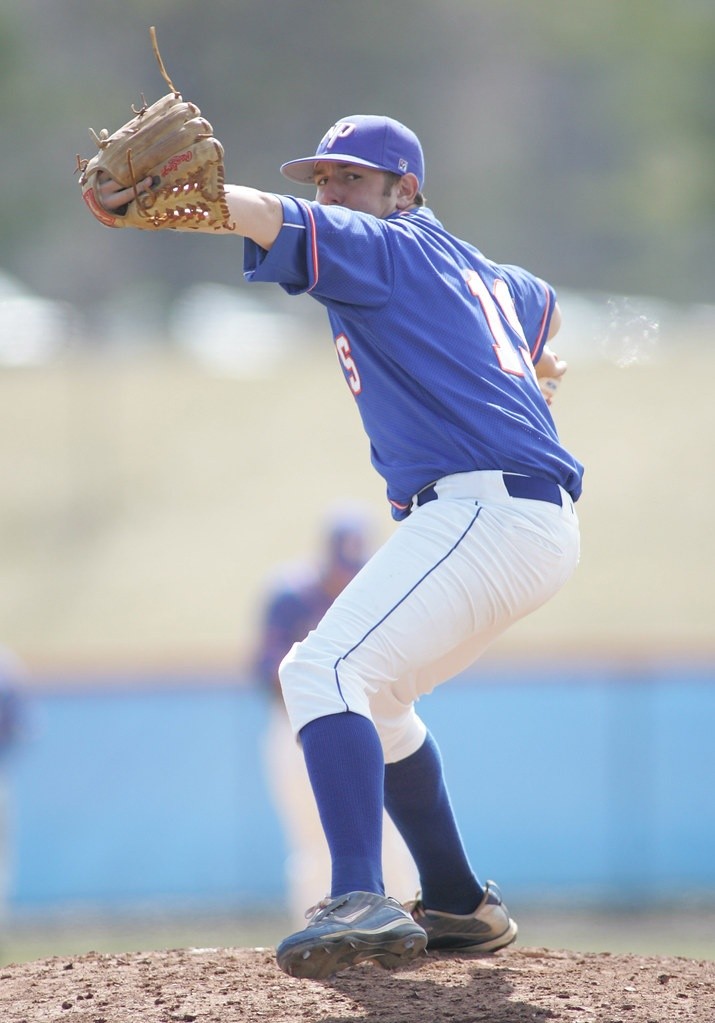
[325,510,367,572]
[280,113,424,194]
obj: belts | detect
[407,474,573,515]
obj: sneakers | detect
[401,880,518,953]
[276,890,428,980]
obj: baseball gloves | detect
[75,91,232,235]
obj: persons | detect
[255,511,422,930]
[83,114,587,973]
